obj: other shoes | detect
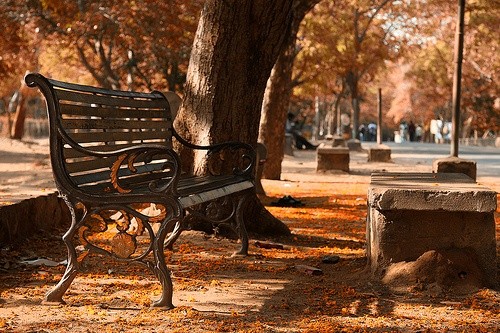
[271,196,304,207]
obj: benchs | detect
[25,72,255,310]
[317,134,392,170]
[366,172,497,274]
[285,132,294,156]
[432,156,477,182]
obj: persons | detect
[434,113,452,144]
[358,121,377,141]
[286,113,320,150]
[399,120,425,143]
